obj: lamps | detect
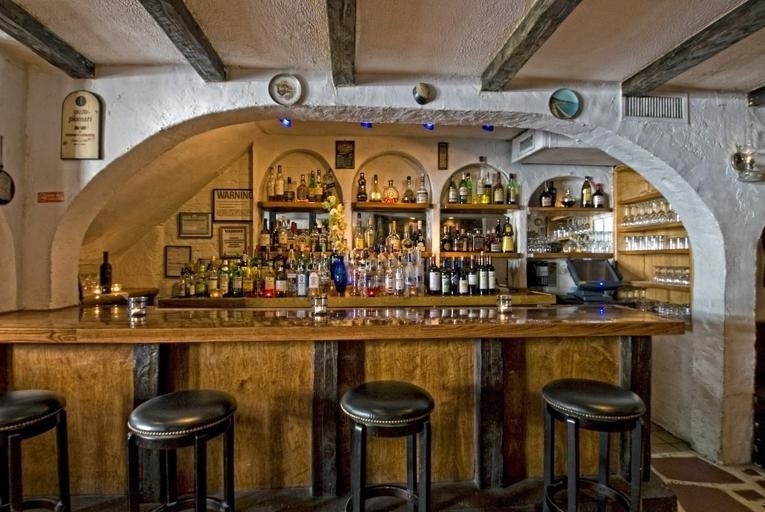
[730,145,764,184]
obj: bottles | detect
[357,171,430,203]
[351,252,497,297]
[99,250,112,294]
[267,164,334,202]
[173,243,347,299]
[538,176,607,207]
[448,172,516,205]
[310,295,327,317]
[354,211,426,252]
[185,310,347,327]
[352,308,497,326]
[442,215,516,253]
[258,217,328,251]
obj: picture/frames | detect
[219,224,248,259]
[211,186,253,224]
[163,244,192,279]
[177,212,212,239]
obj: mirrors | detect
[266,212,329,236]
[532,210,616,252]
[354,211,426,254]
[441,213,516,252]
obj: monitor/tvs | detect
[567,259,624,291]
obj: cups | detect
[499,294,513,313]
[640,300,691,319]
[623,234,690,251]
[655,265,691,286]
[127,295,147,322]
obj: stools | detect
[125,389,238,512]
[340,381,435,512]
[1,389,72,511]
[541,378,646,512]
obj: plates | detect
[267,73,303,104]
[549,89,579,120]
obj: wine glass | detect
[621,198,682,226]
[527,215,613,253]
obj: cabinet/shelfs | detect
[613,165,693,331]
[260,199,337,263]
[546,216,596,243]
[526,206,615,258]
[440,204,521,261]
[352,203,431,261]
